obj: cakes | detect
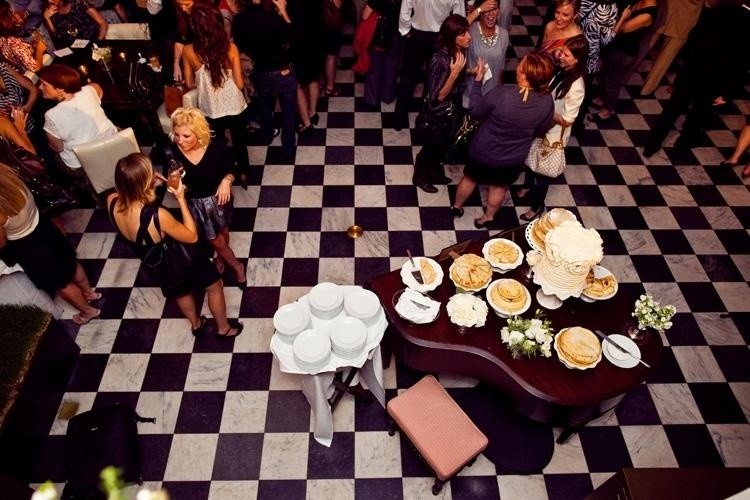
[538,220,605,292]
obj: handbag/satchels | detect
[126,61,151,99]
[524,116,566,179]
[428,97,457,148]
[143,204,192,280]
[7,147,80,215]
[453,113,479,152]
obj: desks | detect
[271,284,389,417]
[362,223,664,444]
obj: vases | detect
[620,313,651,348]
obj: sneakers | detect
[598,107,616,120]
[270,128,280,136]
[592,95,607,106]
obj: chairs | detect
[19,17,202,220]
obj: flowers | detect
[498,305,553,360]
[631,293,676,332]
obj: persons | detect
[586,1,660,124]
[354,0,514,129]
[449,47,555,229]
[410,13,480,196]
[540,0,582,53]
[511,34,591,224]
[621,1,708,102]
[573,0,633,139]
[719,114,750,180]
[641,1,750,166]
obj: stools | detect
[63,402,159,487]
[386,374,491,496]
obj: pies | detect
[451,253,492,289]
[491,279,528,313]
[419,259,437,284]
[583,267,616,297]
[532,208,579,251]
[557,326,602,367]
[489,240,519,264]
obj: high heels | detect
[235,262,247,290]
[83,292,102,305]
[215,323,243,340]
[239,172,249,190]
[216,254,225,276]
[309,113,319,125]
[324,88,341,97]
[473,217,496,230]
[72,308,101,325]
[192,315,207,338]
[449,205,464,223]
[520,206,545,220]
[295,124,314,140]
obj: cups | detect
[166,166,182,193]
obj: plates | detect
[272,281,381,372]
[392,218,642,369]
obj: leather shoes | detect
[742,164,750,178]
[431,173,451,184]
[721,159,739,166]
[412,174,438,193]
